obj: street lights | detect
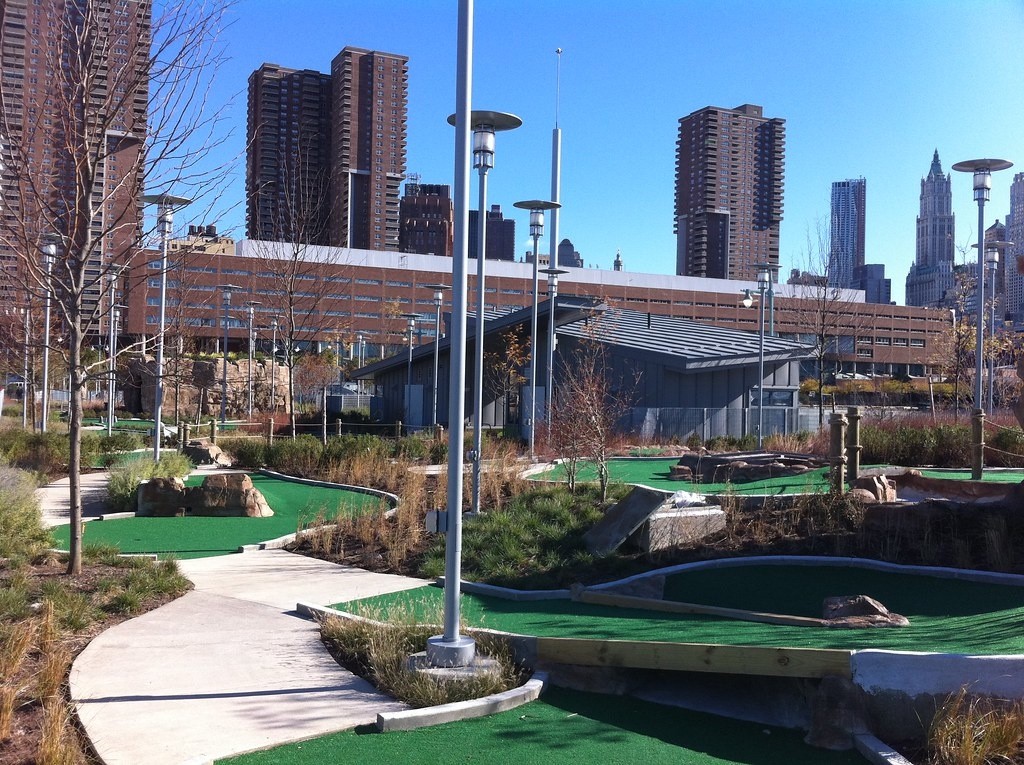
[745,261,784,449]
[40,232,69,433]
[971,241,1015,417]
[424,283,453,426]
[512,198,562,459]
[18,285,43,431]
[107,303,130,427]
[445,107,524,515]
[361,336,372,394]
[100,262,131,437]
[354,330,368,417]
[216,282,244,431]
[136,193,195,467]
[400,311,424,433]
[740,268,777,337]
[540,266,570,445]
[264,314,287,415]
[241,300,262,424]
[950,158,1015,479]
